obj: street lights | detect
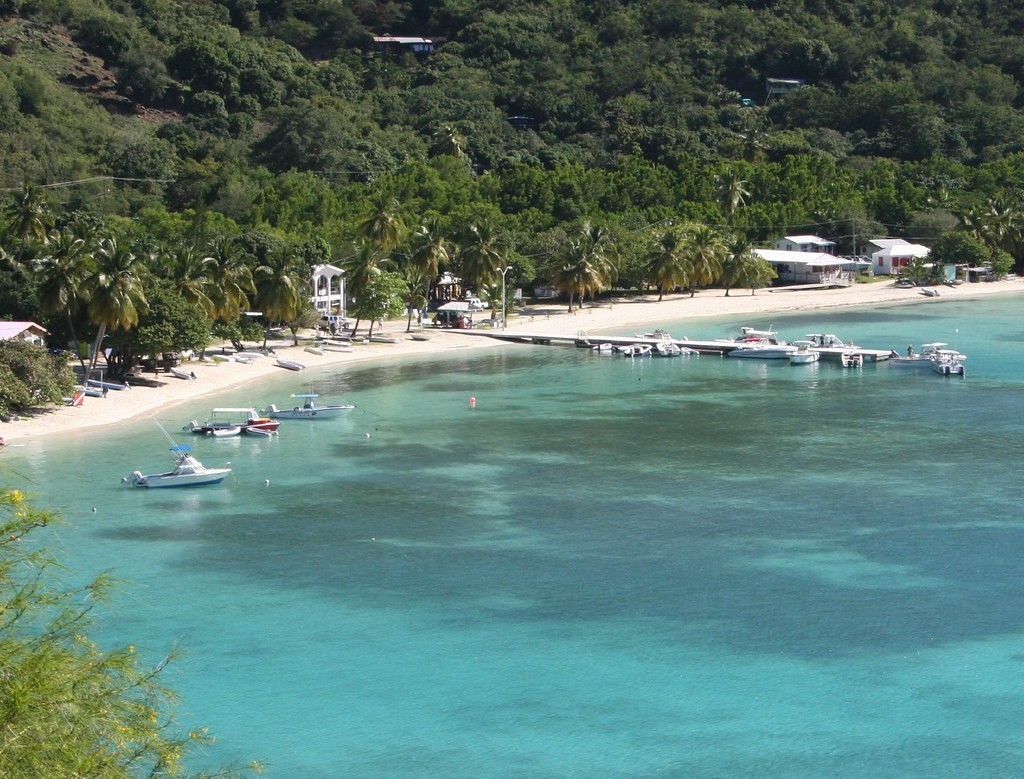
[495,266,514,328]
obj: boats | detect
[323,338,352,347]
[929,351,968,379]
[591,342,612,352]
[617,343,653,355]
[921,287,939,299]
[322,345,353,353]
[804,335,862,350]
[87,379,126,391]
[634,330,680,342]
[841,352,865,369]
[70,387,104,397]
[728,345,799,358]
[303,346,325,357]
[183,407,281,432]
[681,347,702,358]
[786,352,824,363]
[258,393,357,419]
[213,425,242,437]
[366,335,402,344]
[170,366,198,380]
[409,332,430,341]
[120,423,233,489]
[892,343,949,363]
[277,359,306,370]
[716,325,788,347]
[244,427,277,436]
[656,342,682,356]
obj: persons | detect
[103,386,109,398]
[907,344,958,366]
[490,310,497,320]
[377,313,439,331]
[811,333,855,348]
